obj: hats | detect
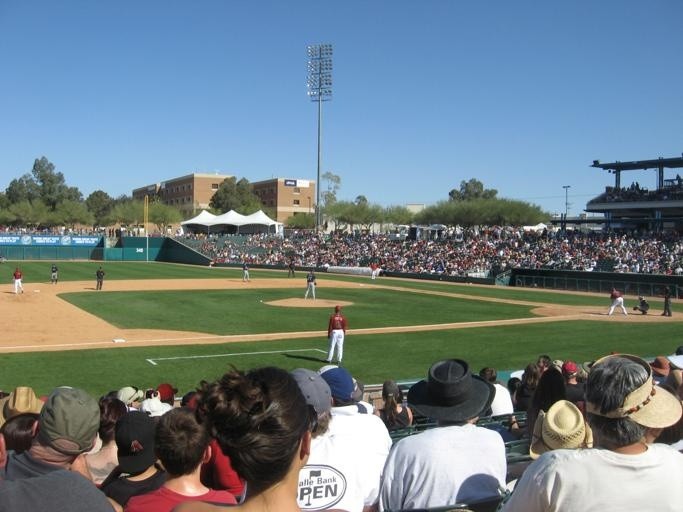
[345,401,346,402]
[0,384,178,475]
[382,381,398,394]
[291,365,364,415]
[407,359,496,422]
[528,353,682,461]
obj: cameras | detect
[146,390,158,399]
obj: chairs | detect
[160,230,683,283]
[361,404,545,510]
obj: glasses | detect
[307,404,318,433]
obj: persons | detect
[0,224,682,283]
[95,266,104,290]
[0,348,682,511]
[304,270,316,300]
[48,263,58,285]
[12,268,23,293]
[632,296,649,315]
[607,287,627,315]
[323,305,345,364]
[661,286,672,316]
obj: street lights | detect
[304,43,335,228]
[562,186,571,215]
